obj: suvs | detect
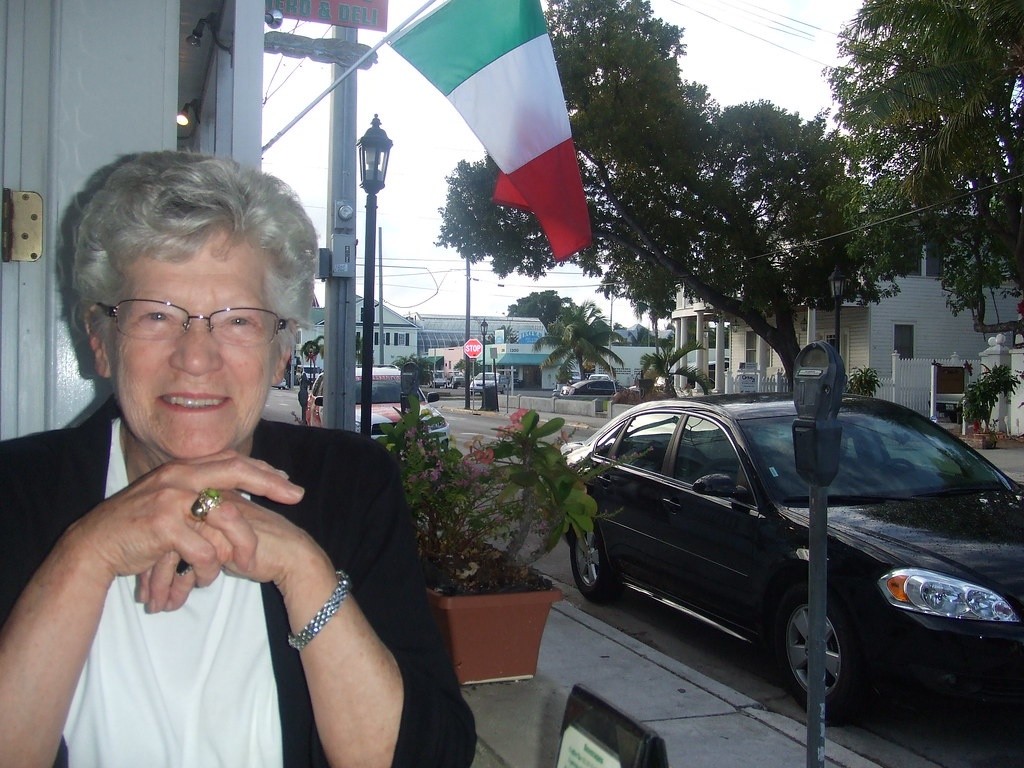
[296,365,321,384]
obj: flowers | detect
[377,399,654,592]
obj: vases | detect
[427,586,565,685]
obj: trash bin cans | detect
[482,386,497,410]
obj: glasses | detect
[96,299,287,349]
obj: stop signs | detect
[464,339,483,358]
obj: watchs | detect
[287,570,353,652]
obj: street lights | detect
[356,113,394,443]
[480,318,488,388]
[828,265,848,353]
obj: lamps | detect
[186,13,233,54]
[177,98,201,126]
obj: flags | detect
[385,0,591,260]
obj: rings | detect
[191,488,222,524]
[176,559,191,576]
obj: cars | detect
[559,379,627,395]
[469,372,510,394]
[305,364,451,452]
[559,391,1024,731]
[589,374,611,380]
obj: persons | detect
[0,153,478,768]
[298,373,310,423]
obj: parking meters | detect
[791,340,848,764]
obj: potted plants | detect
[958,363,1021,449]
